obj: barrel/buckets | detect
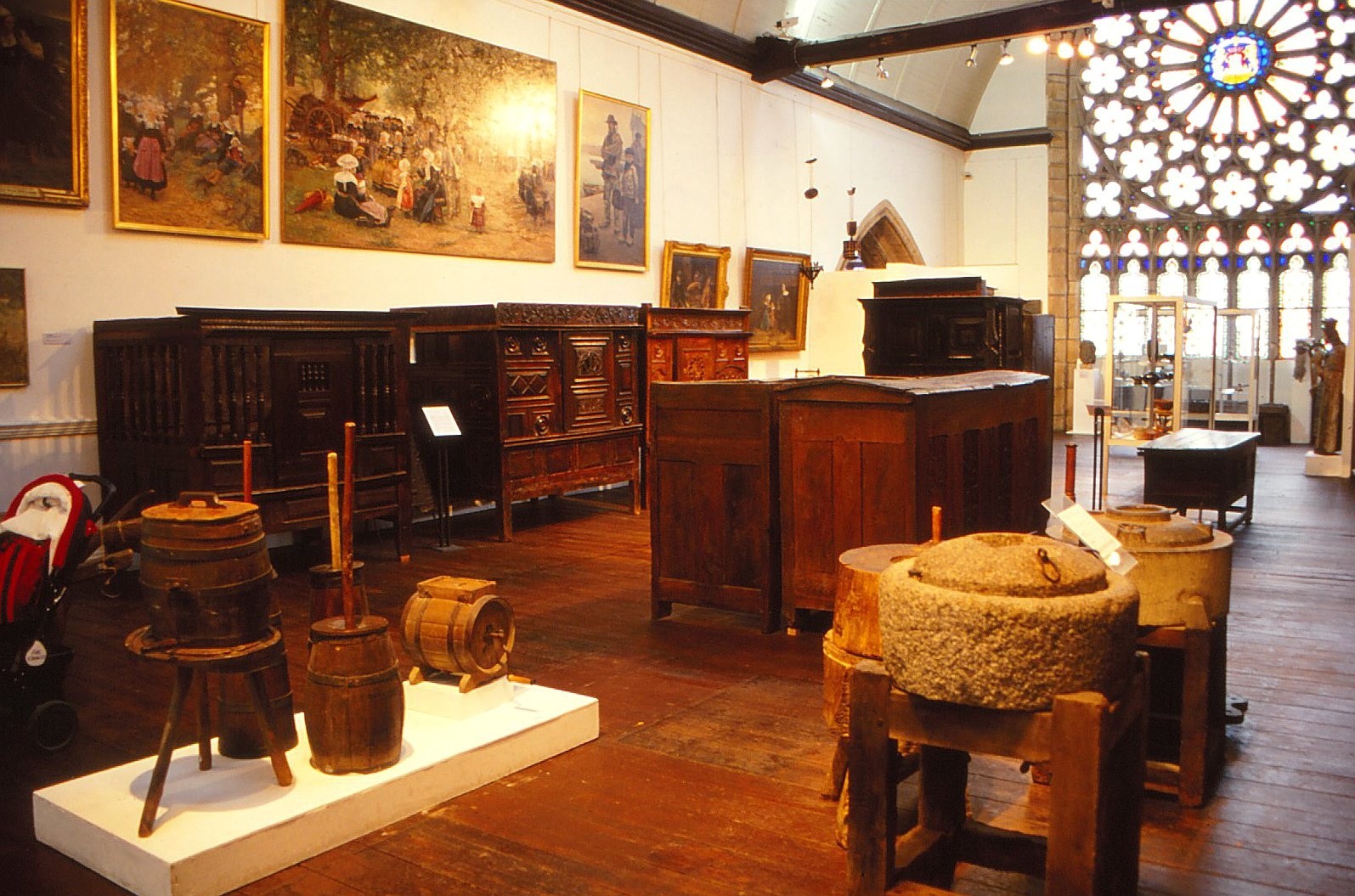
[139,491,275,645]
[305,629,403,773]
[398,592,515,679]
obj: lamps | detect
[1045,28,1096,60]
[820,65,835,90]
[874,58,889,79]
[965,43,976,68]
[998,39,1016,65]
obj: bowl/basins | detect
[1153,399,1173,409]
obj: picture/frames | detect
[109,0,271,242]
[0,266,31,386]
[279,0,556,263]
[743,246,812,351]
[573,89,651,272]
[0,0,90,209]
[660,240,731,310]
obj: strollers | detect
[0,473,116,755]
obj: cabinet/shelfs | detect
[93,304,755,598]
[860,278,1028,376]
[648,367,1050,636]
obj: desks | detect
[1147,406,1188,429]
[1137,427,1261,534]
